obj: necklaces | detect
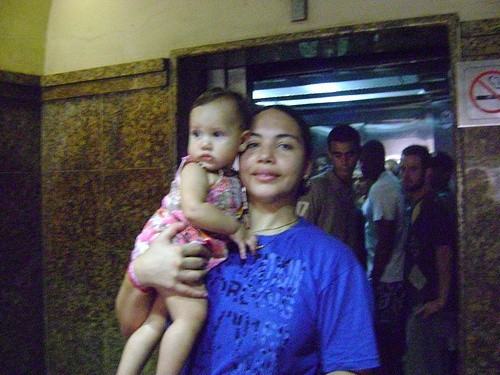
[256,239,274,249]
[253,218,301,232]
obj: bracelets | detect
[128,260,153,293]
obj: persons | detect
[115,87,257,375]
[116,105,380,375]
[295,124,457,375]
